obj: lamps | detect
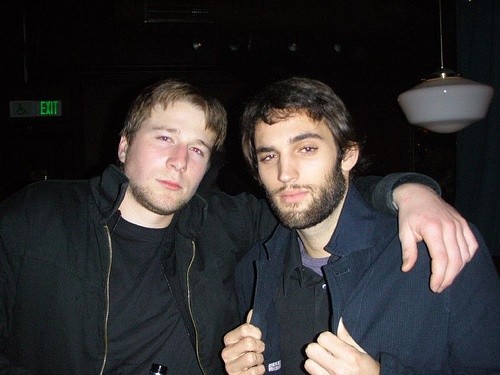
[397,0,494,133]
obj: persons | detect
[220,76,500,375]
[0,76,478,375]
